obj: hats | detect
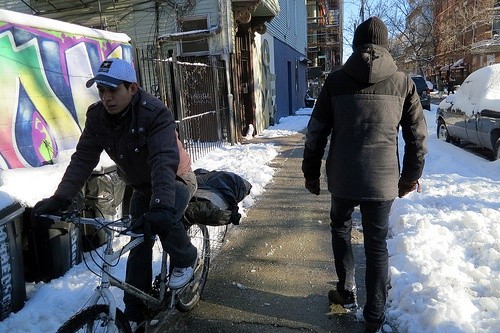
[353,16,388,50]
[86,58,137,90]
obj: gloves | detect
[398,177,420,198]
[150,210,171,240]
[33,197,61,223]
[305,177,320,195]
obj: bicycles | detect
[34,204,211,333]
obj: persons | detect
[437,77,444,96]
[447,76,455,96]
[301,16,428,333]
[32,57,197,332]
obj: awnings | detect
[451,58,464,68]
[440,65,450,70]
[433,65,443,71]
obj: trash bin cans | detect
[0,200,28,322]
[80,164,125,251]
[23,192,87,284]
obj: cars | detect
[435,63,500,163]
[409,73,431,111]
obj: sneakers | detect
[364,316,386,333]
[128,321,137,333]
[169,257,198,289]
[328,289,358,308]
